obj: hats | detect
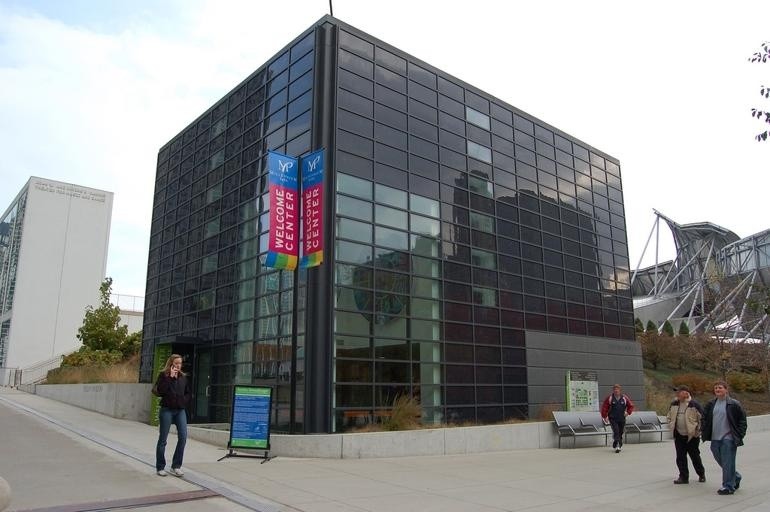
[673,385,689,392]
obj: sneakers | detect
[170,467,183,476]
[615,446,622,452]
[674,479,689,483]
[718,487,734,495]
[735,475,741,489]
[699,468,706,482]
[157,470,167,476]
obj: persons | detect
[601,384,634,453]
[152,354,192,476]
[704,382,747,495]
[667,385,705,483]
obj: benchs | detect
[552,410,674,449]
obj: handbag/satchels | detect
[151,382,160,397]
